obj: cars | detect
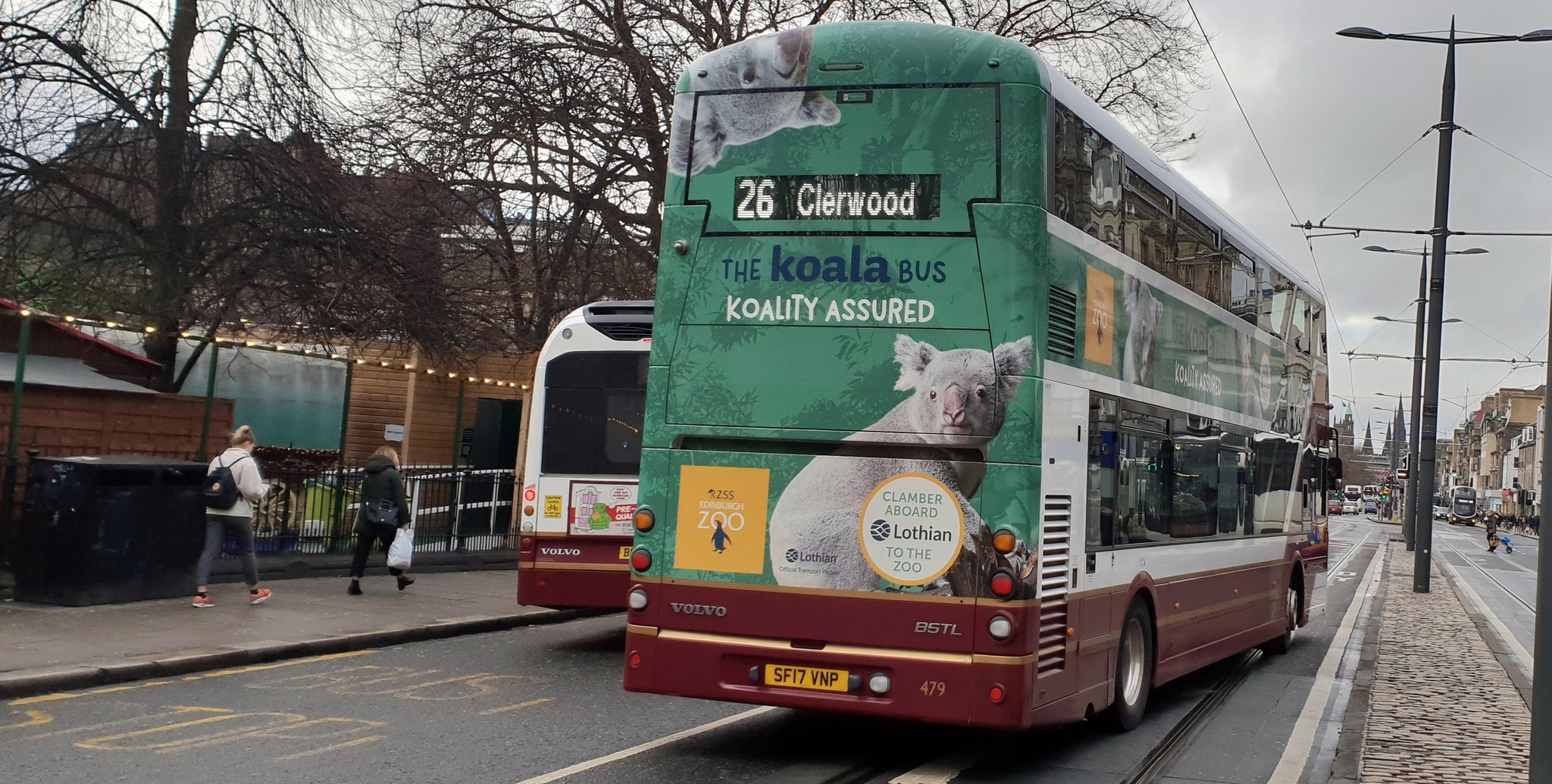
[1343,501,1359,515]
[1328,500,1342,516]
[1364,502,1378,514]
[1433,493,1449,521]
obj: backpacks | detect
[198,457,251,510]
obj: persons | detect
[1477,509,1488,526]
[1487,510,1496,551]
[348,446,416,595]
[1492,511,1540,536]
[193,426,271,607]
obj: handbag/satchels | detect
[365,498,402,528]
[386,528,419,571]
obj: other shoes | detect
[347,584,363,595]
[398,575,417,591]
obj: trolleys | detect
[1496,535,1513,553]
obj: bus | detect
[1345,485,1363,511]
[1363,485,1378,501]
[622,19,1346,736]
[518,299,657,610]
[1450,486,1477,527]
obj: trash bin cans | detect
[13,449,210,607]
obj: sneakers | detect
[249,587,273,605]
[192,595,215,608]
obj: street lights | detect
[1333,26,1552,593]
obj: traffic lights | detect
[1334,490,1339,497]
[1382,494,1387,499]
[1402,455,1406,469]
[1342,490,1345,496]
[1396,451,1420,483]
[1386,476,1392,489]
